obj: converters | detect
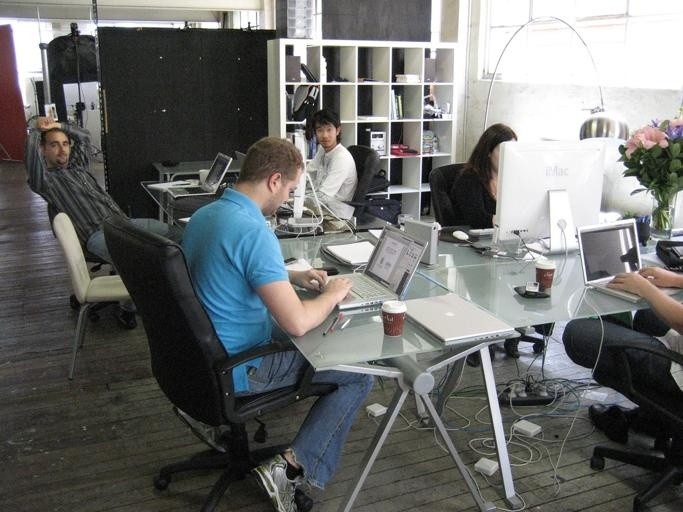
[366,403,387,419]
[474,457,499,475]
[513,419,543,437]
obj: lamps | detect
[484,13,631,144]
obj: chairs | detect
[587,337,683,512]
[50,209,130,378]
[104,223,335,512]
[427,160,546,367]
[44,201,125,322]
[341,142,377,219]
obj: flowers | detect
[614,108,681,209]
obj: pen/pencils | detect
[323,313,353,335]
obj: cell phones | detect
[525,281,540,293]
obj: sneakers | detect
[251,454,304,512]
[172,405,227,453]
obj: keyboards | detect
[470,227,492,237]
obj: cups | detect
[381,300,408,337]
[536,259,555,288]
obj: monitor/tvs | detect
[497,139,605,254]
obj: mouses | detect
[452,230,468,240]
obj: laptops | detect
[234,151,248,170]
[402,292,515,346]
[318,226,427,312]
[167,153,233,199]
[581,218,681,302]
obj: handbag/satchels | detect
[366,198,402,225]
[366,169,390,193]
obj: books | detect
[391,89,404,121]
[396,74,420,84]
[147,180,194,193]
[287,128,317,160]
[320,239,377,267]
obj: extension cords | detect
[498,390,555,406]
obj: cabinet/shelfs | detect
[264,40,463,223]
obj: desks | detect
[417,241,681,508]
[151,157,246,228]
[138,175,392,240]
[265,229,521,512]
[355,198,674,423]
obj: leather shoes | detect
[652,432,682,470]
[467,350,481,367]
[118,308,137,329]
[587,403,631,443]
[504,336,520,358]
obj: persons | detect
[25,116,140,329]
[451,122,519,366]
[183,138,376,512]
[563,266,683,438]
[295,108,359,230]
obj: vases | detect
[646,189,674,239]
